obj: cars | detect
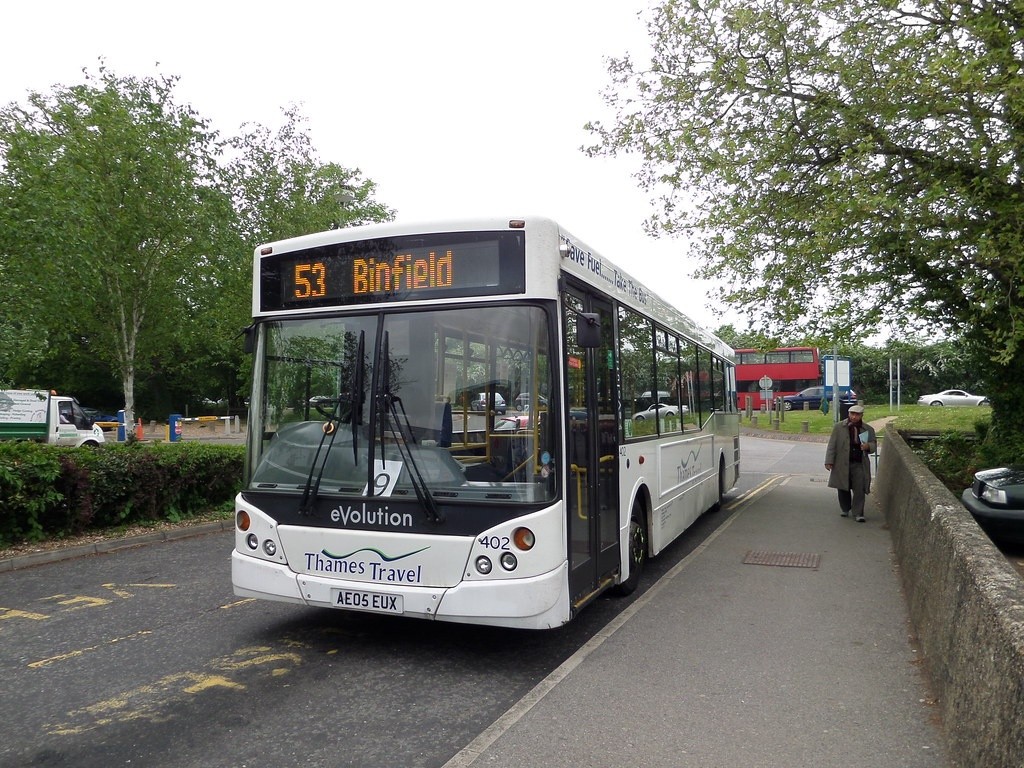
[916,389,990,407]
[959,465,1024,551]
[303,396,333,408]
[570,408,588,421]
[631,403,689,421]
[62,406,118,431]
[494,416,541,429]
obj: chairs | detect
[513,415,617,475]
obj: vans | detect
[642,390,671,405]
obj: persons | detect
[824,405,877,523]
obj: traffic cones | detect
[135,418,149,441]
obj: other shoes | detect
[841,511,848,516]
[855,516,865,522]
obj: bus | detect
[733,347,820,411]
[230,215,741,633]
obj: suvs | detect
[514,392,549,412]
[773,385,859,411]
[470,392,507,415]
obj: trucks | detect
[0,387,106,449]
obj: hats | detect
[848,405,864,414]
